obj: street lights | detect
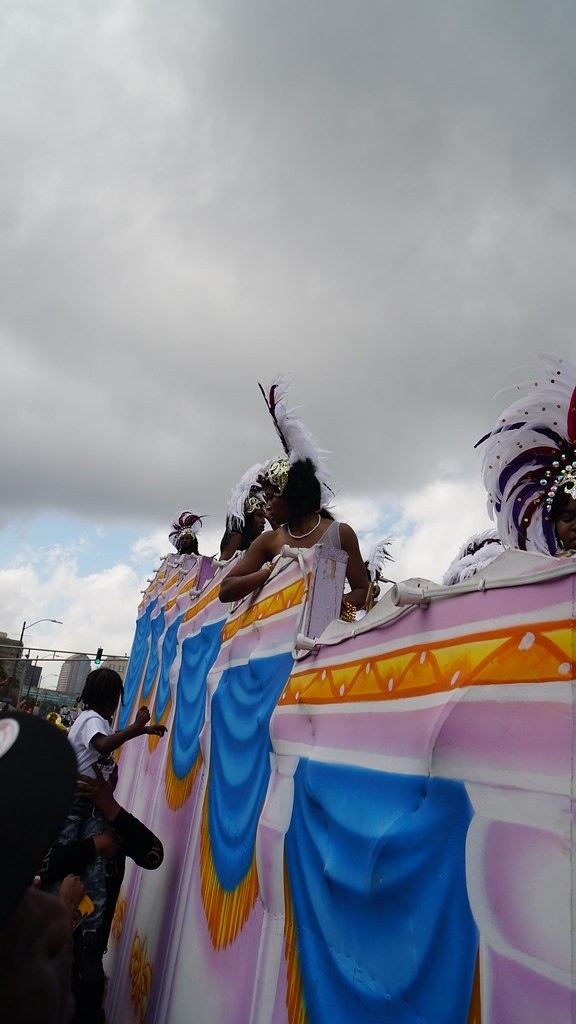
[35,673,59,706]
[26,655,61,703]
[4,618,64,712]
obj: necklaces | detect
[288,514,321,538]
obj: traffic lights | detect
[25,703,35,715]
[95,648,105,664]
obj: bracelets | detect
[262,562,271,577]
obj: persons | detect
[443,352,576,587]
[168,507,213,556]
[218,380,379,624]
[0,667,168,1024]
